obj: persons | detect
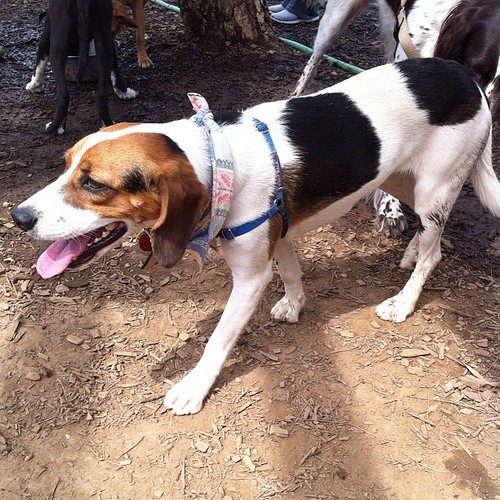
[268,0,320,23]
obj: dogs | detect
[287,0,500,232]
[11,57,500,416]
[25,0,153,135]
